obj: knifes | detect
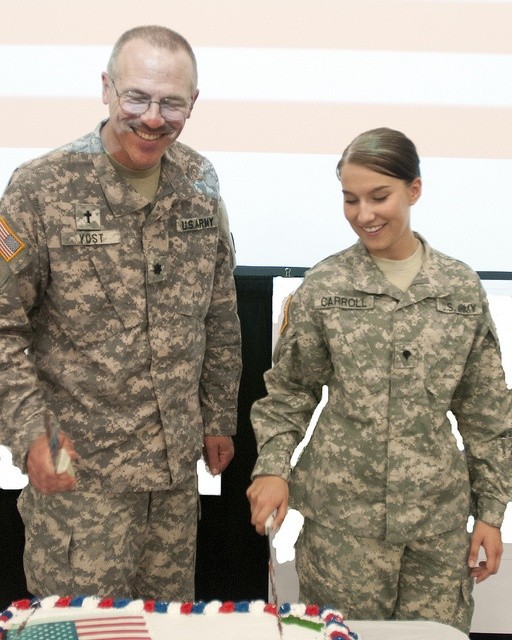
[262,506,286,639]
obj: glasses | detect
[109,77,193,121]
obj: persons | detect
[0,26,242,602]
[246,128,512,635]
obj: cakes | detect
[0,595,356,640]
